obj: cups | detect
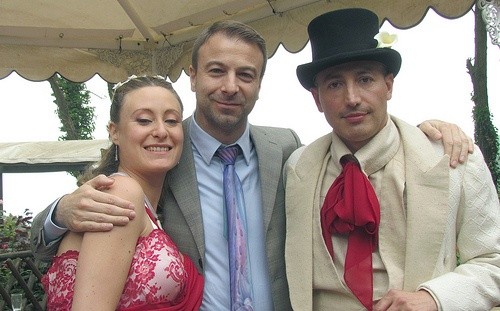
[11,294,22,311]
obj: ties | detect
[214,146,256,311]
[319,161,382,311]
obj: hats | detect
[296,8,401,91]
